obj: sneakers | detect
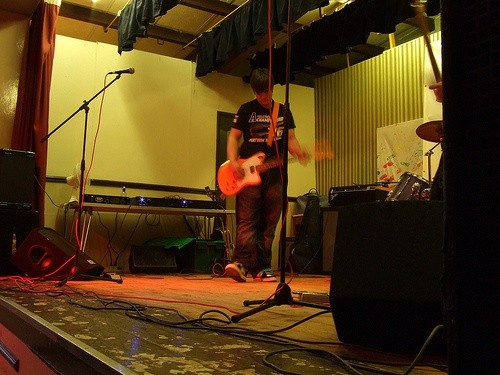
[225,260,248,282]
[253,268,277,282]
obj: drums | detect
[384,170,432,201]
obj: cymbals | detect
[416,119,443,143]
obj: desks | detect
[64,202,236,256]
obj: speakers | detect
[129,245,177,274]
[0,148,104,281]
[321,0,500,375]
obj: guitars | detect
[217,150,335,196]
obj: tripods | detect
[230,0,333,326]
[40,72,123,288]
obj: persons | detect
[429,81,443,201]
[224,68,311,282]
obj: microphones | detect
[109,68,136,74]
